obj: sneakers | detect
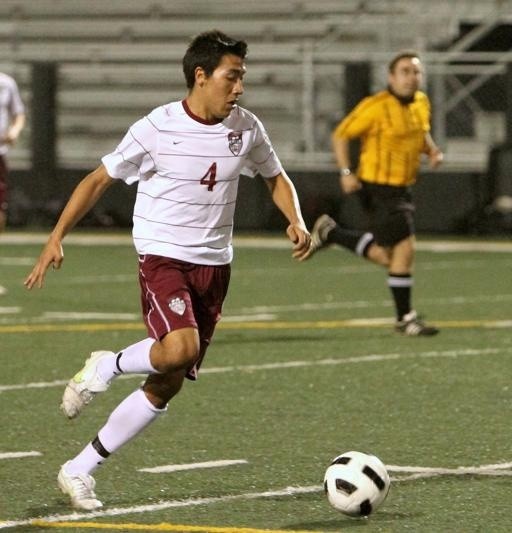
[309,215,337,255]
[57,460,102,510]
[61,351,111,419]
[394,309,437,336]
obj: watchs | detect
[338,168,353,176]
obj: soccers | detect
[325,450,390,519]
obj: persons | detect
[310,53,443,336]
[0,73,27,233]
[24,29,316,510]
